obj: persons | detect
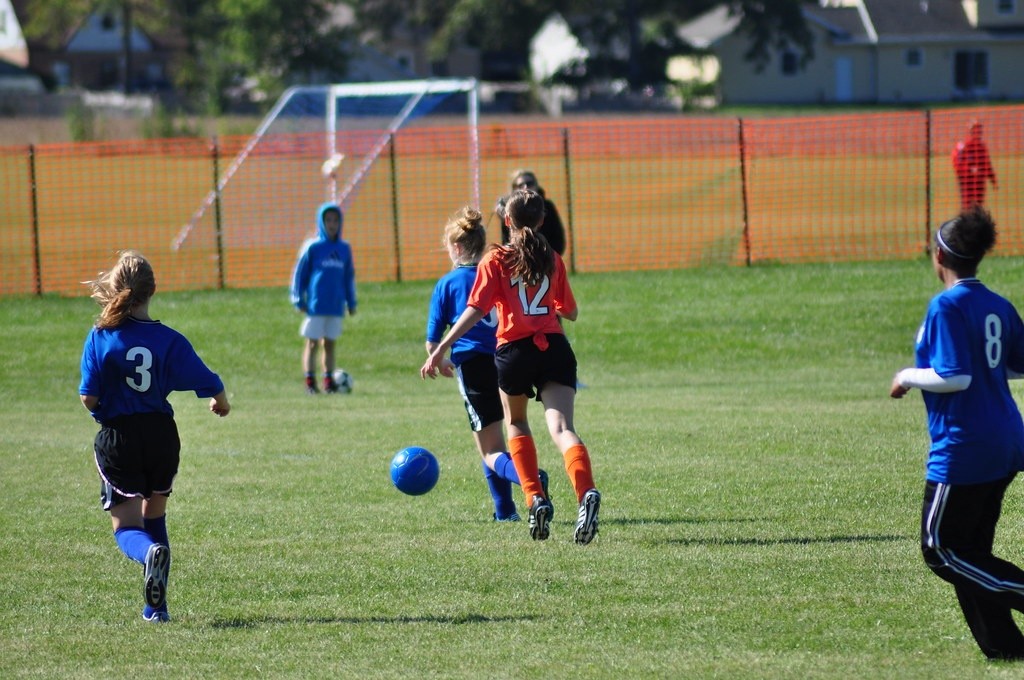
[421,190,602,544]
[80,251,230,621]
[496,170,565,257]
[291,202,356,394]
[889,210,1023,662]
[951,119,998,211]
[425,208,554,524]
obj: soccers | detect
[389,447,440,496]
[332,370,353,394]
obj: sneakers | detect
[142,600,169,623]
[527,493,551,541]
[574,488,601,546]
[143,542,170,610]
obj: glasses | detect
[518,181,534,190]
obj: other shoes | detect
[538,468,554,523]
[325,388,334,395]
[492,512,522,523]
[307,387,320,397]
